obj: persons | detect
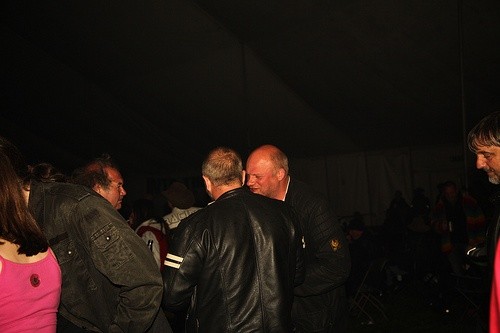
[467,112,500,333]
[0,144,63,333]
[243,144,352,333]
[119,188,168,270]
[346,219,375,250]
[163,182,205,229]
[434,181,485,241]
[0,139,163,333]
[70,158,126,210]
[165,146,306,333]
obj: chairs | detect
[351,253,390,324]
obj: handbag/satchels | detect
[136,217,169,274]
